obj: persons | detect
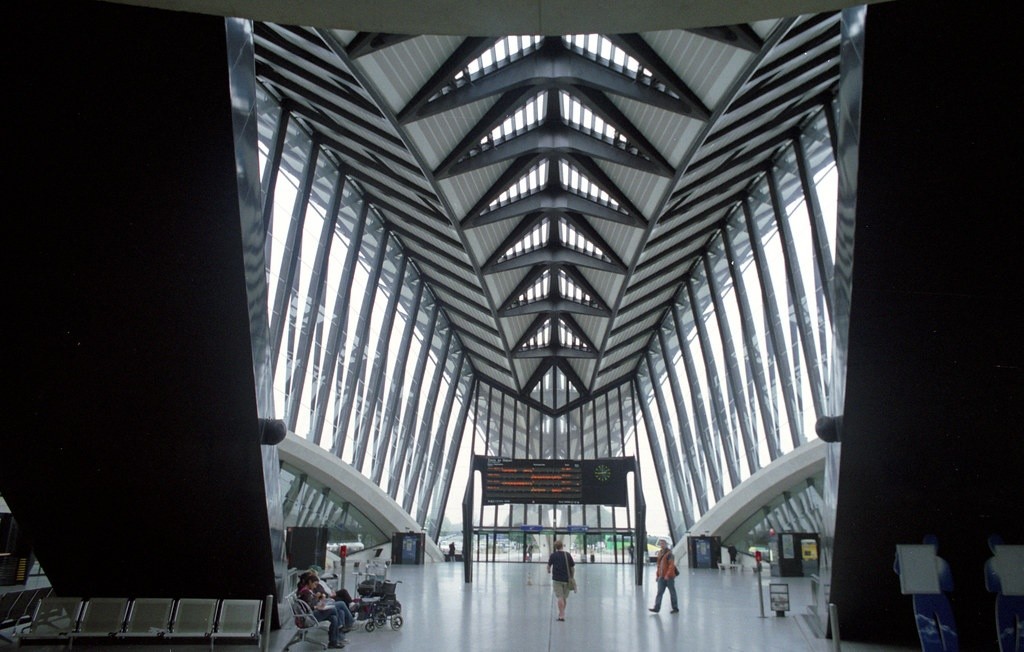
[294,569,360,649]
[547,540,575,622]
[728,543,737,569]
[628,543,634,564]
[528,543,534,562]
[449,542,455,562]
[648,539,679,613]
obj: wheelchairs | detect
[348,574,403,633]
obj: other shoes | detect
[348,621,365,630]
[327,643,345,649]
[340,627,350,634]
[350,603,360,611]
[558,617,564,622]
[670,608,679,614]
[338,638,350,643]
[649,609,659,612]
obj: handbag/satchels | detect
[568,578,578,593]
[357,579,383,597]
[674,566,680,577]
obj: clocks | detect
[594,465,611,481]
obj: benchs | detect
[0,586,63,644]
[718,563,744,572]
[11,595,274,652]
[284,587,332,651]
